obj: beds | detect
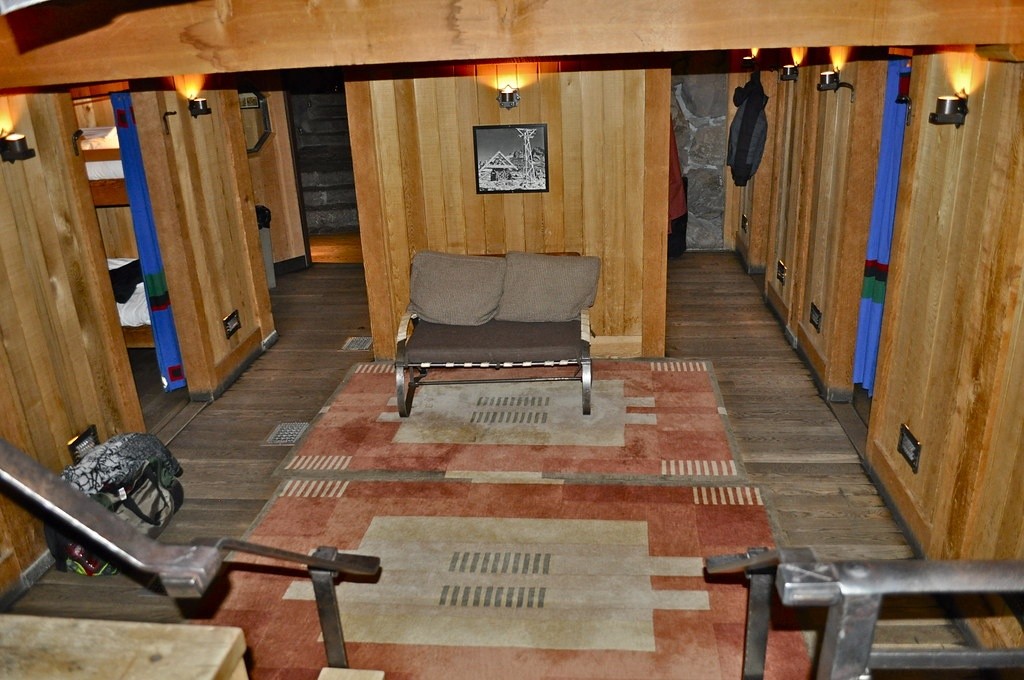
[79,125,186,395]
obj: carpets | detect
[183,478,814,680]
[271,359,749,483]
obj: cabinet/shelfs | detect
[288,90,359,235]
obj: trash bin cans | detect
[255,205,277,288]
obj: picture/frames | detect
[472,124,550,194]
[240,93,260,109]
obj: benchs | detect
[394,250,601,418]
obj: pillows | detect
[494,250,600,322]
[406,251,506,327]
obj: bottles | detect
[50,529,100,575]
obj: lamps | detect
[741,55,762,73]
[188,95,212,119]
[815,66,855,102]
[927,88,968,129]
[0,132,37,164]
[780,61,800,84]
[496,85,522,111]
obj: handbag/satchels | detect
[89,457,185,540]
